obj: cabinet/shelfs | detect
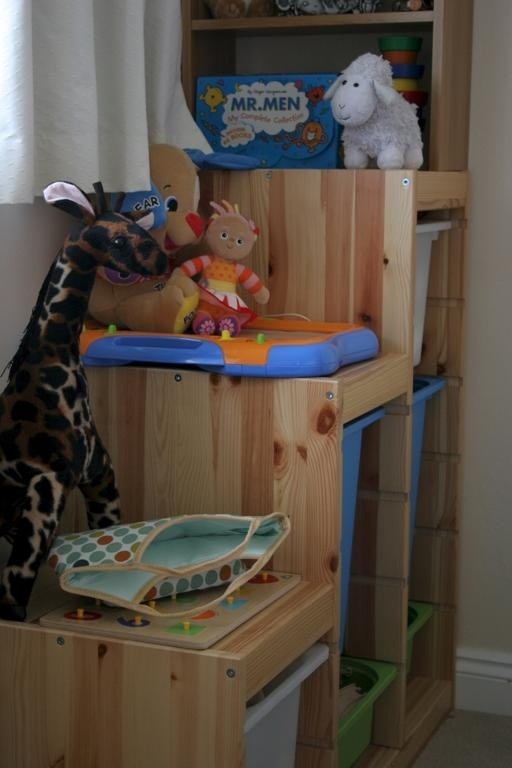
[0,1,477,767]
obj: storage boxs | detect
[407,374,447,588]
[414,220,453,367]
[243,641,329,768]
[407,600,434,675]
[339,408,387,656]
[338,654,398,768]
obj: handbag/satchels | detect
[48,512,292,621]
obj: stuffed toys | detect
[88,142,202,335]
[325,51,424,171]
[179,198,271,321]
[1,184,163,623]
